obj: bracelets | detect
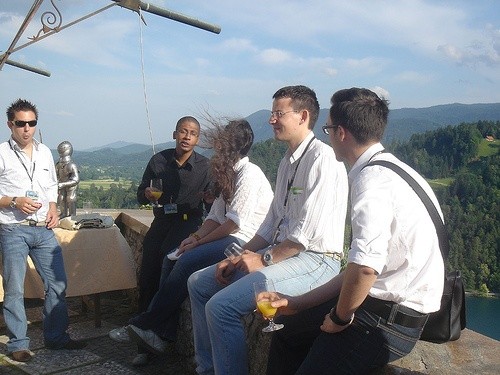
[191,234,200,241]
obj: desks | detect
[0,223,139,330]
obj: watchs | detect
[9,197,18,209]
[262,250,273,266]
[329,306,353,326]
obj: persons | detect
[55,141,79,219]
[255,87,444,375]
[110,119,274,355]
[0,99,85,362]
[131,116,218,366]
[187,85,351,375]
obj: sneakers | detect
[109,325,134,342]
[133,353,147,365]
[126,324,169,355]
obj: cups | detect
[224,241,245,264]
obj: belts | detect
[18,221,48,227]
[325,252,343,261]
[365,298,426,328]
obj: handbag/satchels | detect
[419,269,467,344]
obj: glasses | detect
[270,110,299,118]
[321,124,347,135]
[11,120,37,127]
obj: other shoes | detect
[49,338,88,349]
[12,348,35,363]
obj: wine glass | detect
[150,178,164,208]
[253,279,284,332]
